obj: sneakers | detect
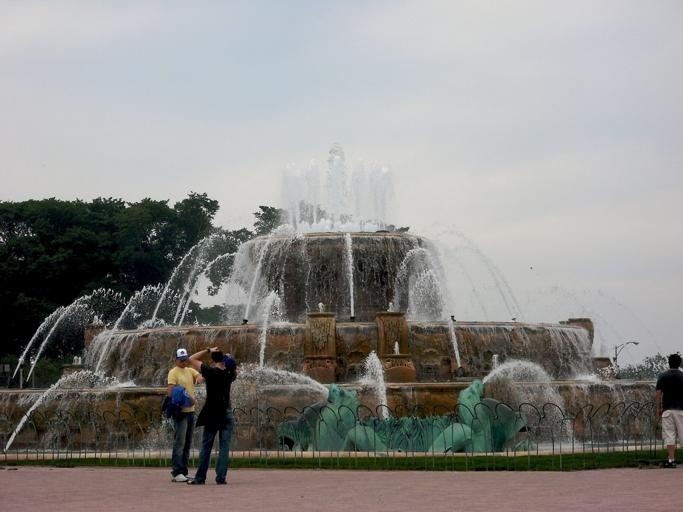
[168,473,190,482]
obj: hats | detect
[174,347,189,360]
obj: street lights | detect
[613,340,640,378]
[29,355,35,389]
[17,357,24,388]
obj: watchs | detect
[207,347,211,354]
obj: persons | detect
[186,345,238,486]
[655,354,682,469]
[167,348,206,482]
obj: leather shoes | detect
[215,480,228,485]
[187,479,206,484]
